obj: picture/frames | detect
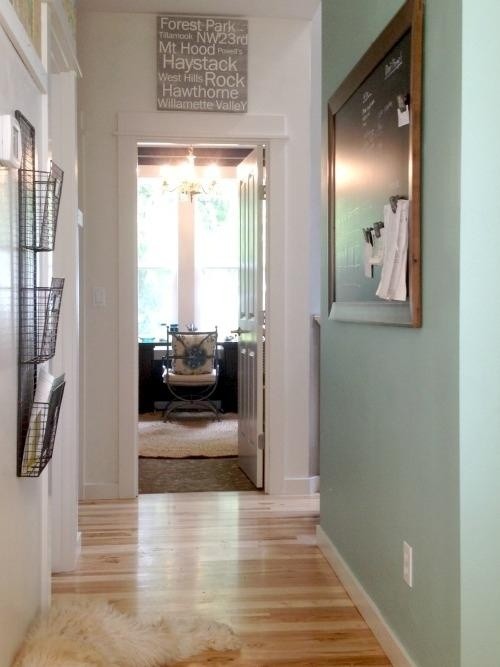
[0,0,54,92]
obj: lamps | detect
[143,147,235,202]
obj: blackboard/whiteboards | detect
[155,15,248,114]
[327,0,421,328]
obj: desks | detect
[154,345,225,409]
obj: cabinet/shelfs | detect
[15,110,66,477]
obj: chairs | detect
[163,326,224,423]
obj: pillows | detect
[172,335,216,375]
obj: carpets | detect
[138,411,237,458]
